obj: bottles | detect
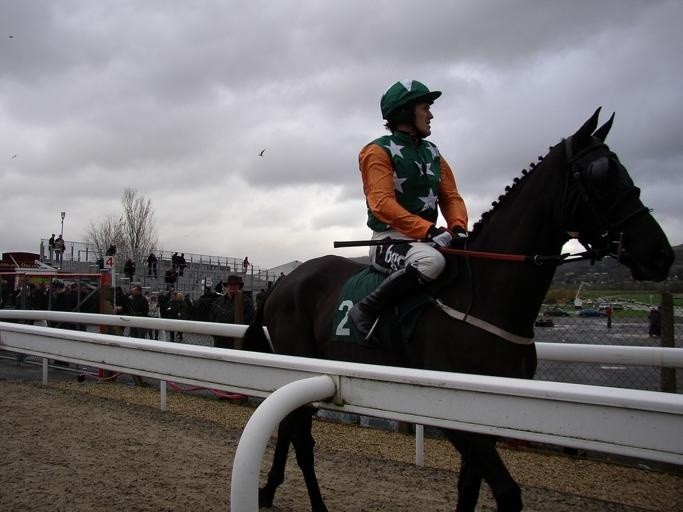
[221,274,244,289]
[379,79,442,120]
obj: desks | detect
[61,212,65,234]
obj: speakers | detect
[346,263,424,344]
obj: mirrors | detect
[256,147,265,158]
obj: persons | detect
[348,80,468,345]
[606,303,614,328]
[0,234,266,352]
[646,305,662,337]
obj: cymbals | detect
[575,280,593,309]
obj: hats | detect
[598,303,608,310]
[544,306,569,317]
[579,309,607,318]
[612,303,623,310]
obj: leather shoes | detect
[427,225,454,249]
[451,224,469,241]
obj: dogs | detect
[236,105,676,512]
